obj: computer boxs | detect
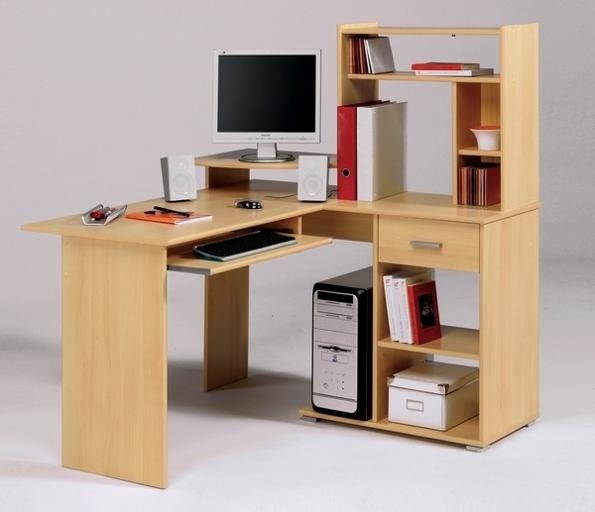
[311,264,373,420]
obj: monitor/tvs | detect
[212,50,322,163]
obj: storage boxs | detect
[387,359,479,431]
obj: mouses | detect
[236,199,262,209]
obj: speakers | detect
[297,154,329,203]
[160,154,197,203]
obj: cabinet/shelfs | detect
[336,22,540,212]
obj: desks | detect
[20,178,543,489]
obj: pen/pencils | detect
[143,205,194,217]
[90,207,119,220]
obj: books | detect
[410,61,493,77]
[126,209,213,224]
[383,271,441,345]
[348,36,395,74]
[458,163,500,205]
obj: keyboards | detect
[191,229,297,263]
